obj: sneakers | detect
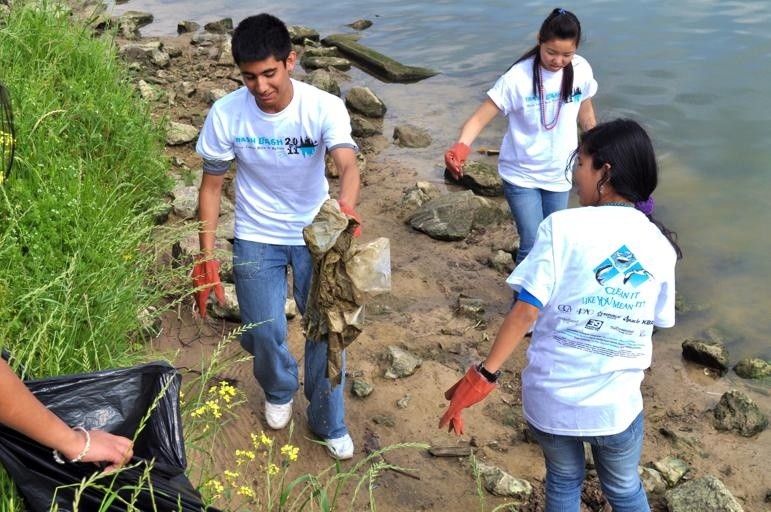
[263,393,295,431]
[315,421,354,460]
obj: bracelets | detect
[53,424,92,466]
[477,360,504,383]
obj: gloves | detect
[193,254,225,318]
[337,199,362,237]
[444,142,473,180]
[438,365,495,438]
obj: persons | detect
[190,13,361,462]
[445,7,602,339]
[0,84,138,475]
[438,116,682,511]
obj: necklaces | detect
[600,200,637,209]
[537,60,566,127]
[539,63,564,130]
